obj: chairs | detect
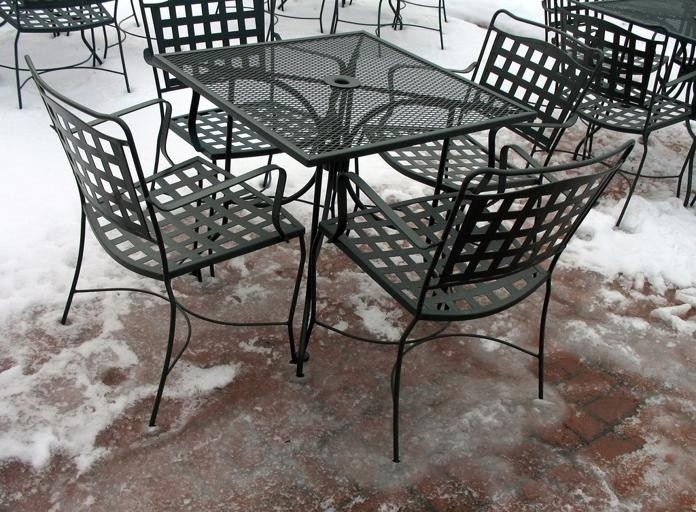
[525,0,696,227]
[345,9,603,281]
[25,55,307,427]
[302,139,635,462]
[0,0,139,110]
[139,0,323,277]
[215,0,447,56]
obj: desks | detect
[144,31,538,378]
